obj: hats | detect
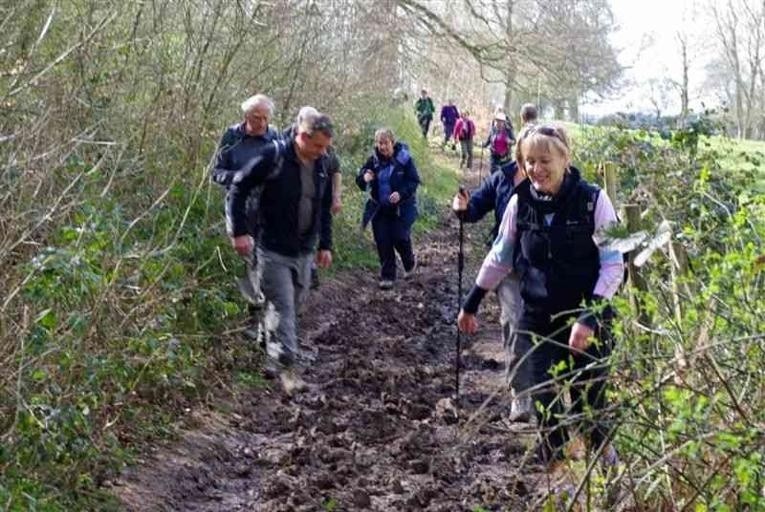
[495,112,506,122]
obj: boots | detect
[242,303,261,339]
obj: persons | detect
[210,92,285,347]
[413,89,538,175]
[457,125,625,479]
[453,126,535,422]
[356,128,419,288]
[283,106,342,291]
[224,113,335,398]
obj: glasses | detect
[516,126,570,152]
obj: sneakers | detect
[280,371,310,397]
[402,257,418,279]
[596,444,617,478]
[260,360,279,379]
[378,279,394,290]
[509,397,533,422]
